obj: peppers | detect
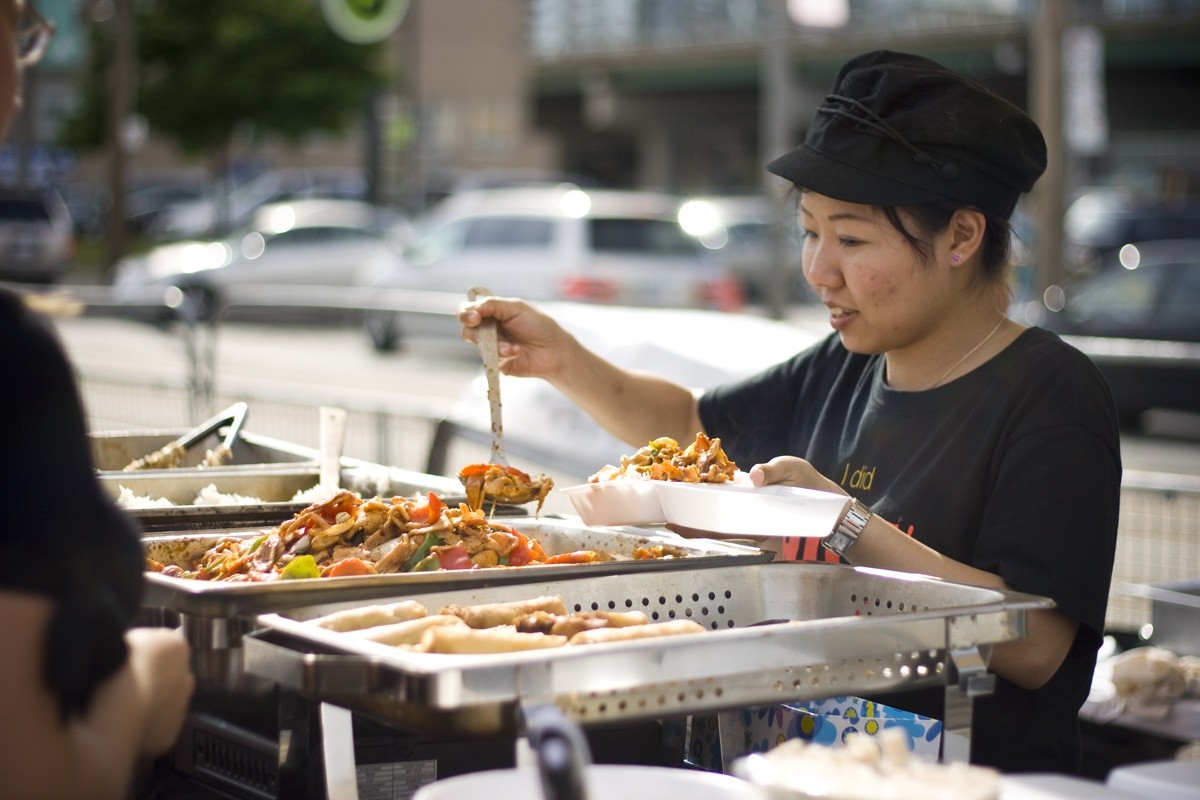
[145,492,594,580]
[459,463,531,483]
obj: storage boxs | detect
[725,697,938,764]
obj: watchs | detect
[820,496,872,557]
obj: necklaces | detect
[927,312,1005,389]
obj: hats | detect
[765,52,1047,208]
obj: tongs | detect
[123,402,249,470]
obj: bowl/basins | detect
[408,763,763,800]
[559,465,849,538]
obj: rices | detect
[115,484,362,510]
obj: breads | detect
[1109,645,1200,704]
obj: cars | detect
[71,164,806,353]
[1041,243,1200,345]
[0,175,106,285]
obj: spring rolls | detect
[293,595,710,654]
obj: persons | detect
[457,47,1122,775]
[0,0,193,800]
